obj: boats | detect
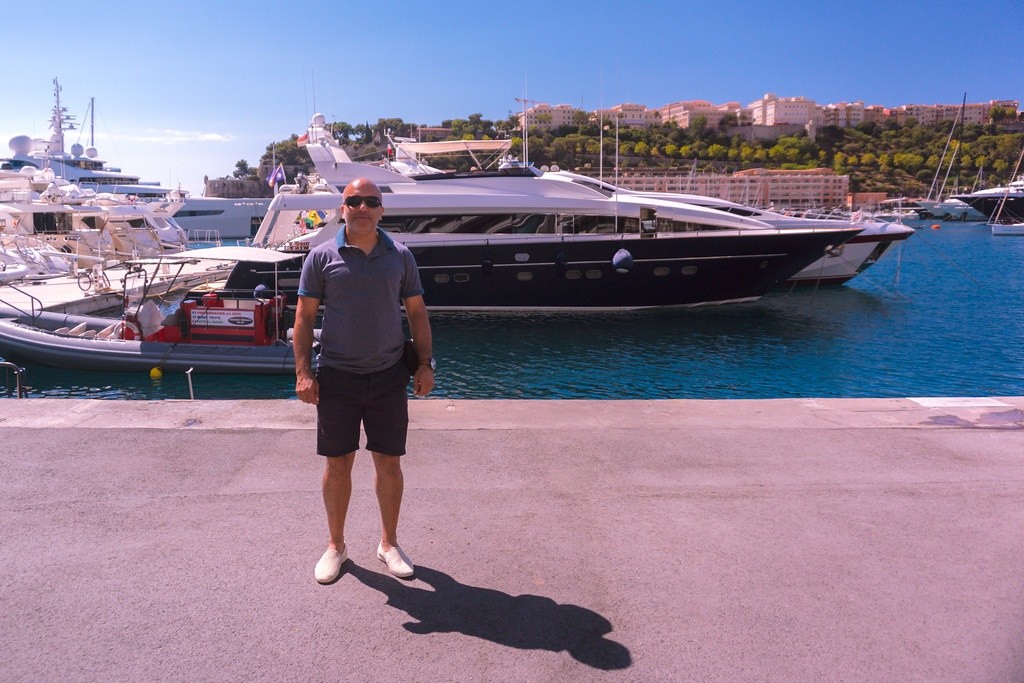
[72,184,190,258]
[2,159,126,271]
[378,136,913,287]
[917,90,1023,235]
[0,241,323,377]
[256,112,867,317]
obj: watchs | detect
[417,357,437,370]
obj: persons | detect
[292,177,439,585]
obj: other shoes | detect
[376,540,416,578]
[314,543,348,584]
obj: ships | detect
[2,75,275,239]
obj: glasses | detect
[342,195,383,209]
